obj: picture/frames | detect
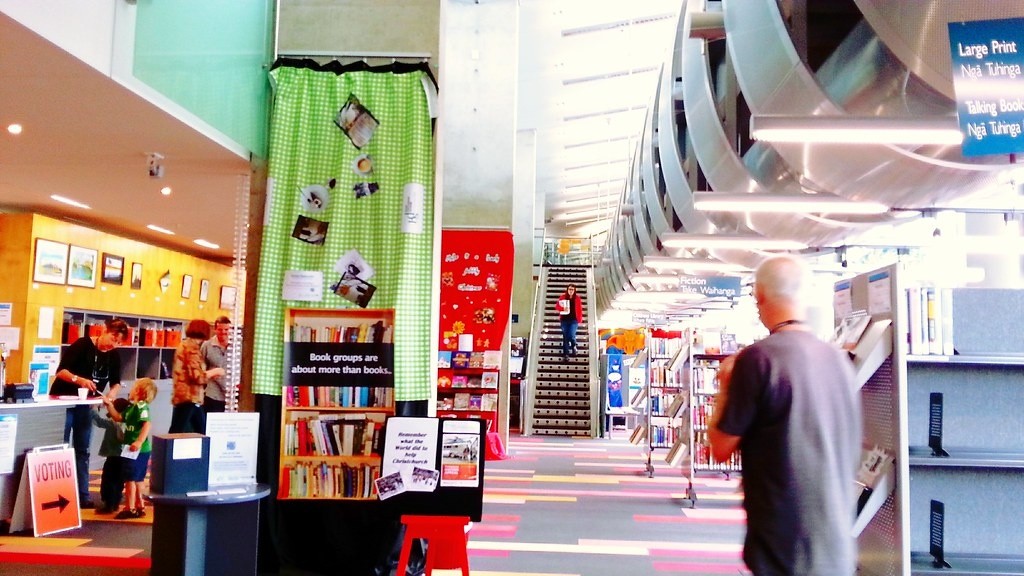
[33,238,236,310]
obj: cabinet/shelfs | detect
[65,307,184,381]
[837,266,1024,576]
[440,368,497,432]
[281,307,394,501]
[633,327,744,507]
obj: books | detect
[627,346,648,446]
[720,333,738,355]
[278,386,394,497]
[558,299,570,315]
[651,336,685,448]
[437,350,503,411]
[280,321,393,344]
[905,287,955,355]
[692,357,742,471]
[664,440,686,468]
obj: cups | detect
[78,388,89,399]
[721,333,739,355]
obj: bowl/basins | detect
[705,347,719,353]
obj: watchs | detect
[72,375,79,382]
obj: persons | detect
[705,257,864,576]
[555,284,582,361]
[48,318,129,507]
[169,320,226,435]
[200,315,232,412]
[94,378,158,519]
[89,398,134,513]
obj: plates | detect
[59,396,80,399]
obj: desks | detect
[608,407,640,439]
[141,483,270,576]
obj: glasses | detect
[569,288,573,290]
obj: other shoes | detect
[572,345,578,355]
[97,499,120,515]
[136,506,146,517]
[79,499,97,509]
[115,508,139,518]
[562,349,569,358]
